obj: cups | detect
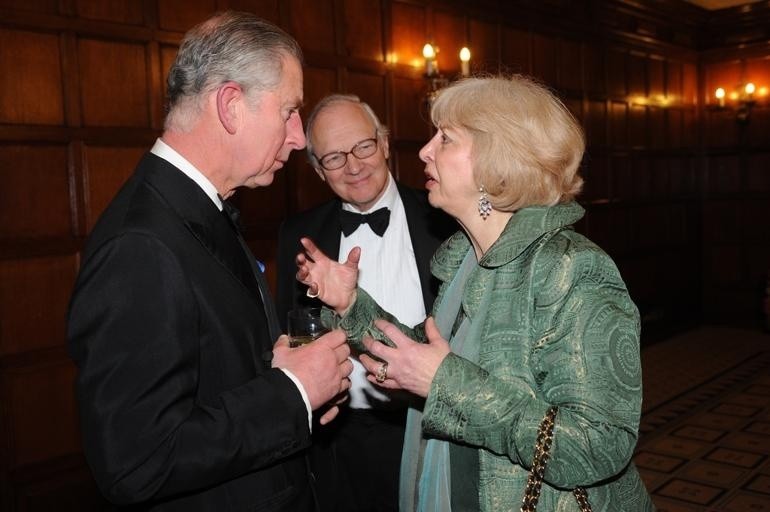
[288,306,338,348]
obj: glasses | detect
[311,127,379,171]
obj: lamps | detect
[422,43,472,106]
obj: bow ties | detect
[337,207,391,237]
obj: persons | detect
[61,11,356,511]
[291,74,662,510]
[271,92,462,512]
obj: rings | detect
[305,287,321,300]
[374,364,388,383]
